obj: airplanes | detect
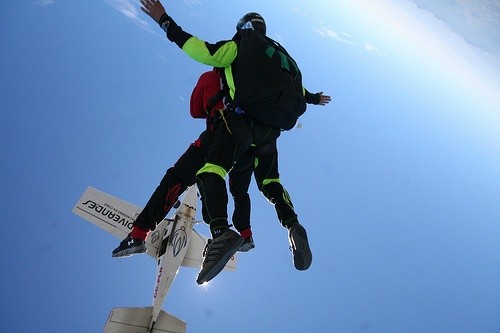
[72,182,237,333]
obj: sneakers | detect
[112,232,147,257]
[236,235,255,252]
[197,228,244,285]
[287,224,312,270]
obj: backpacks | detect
[238,28,306,131]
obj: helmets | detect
[236,12,266,36]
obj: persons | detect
[140,0,332,285]
[111,66,256,258]
